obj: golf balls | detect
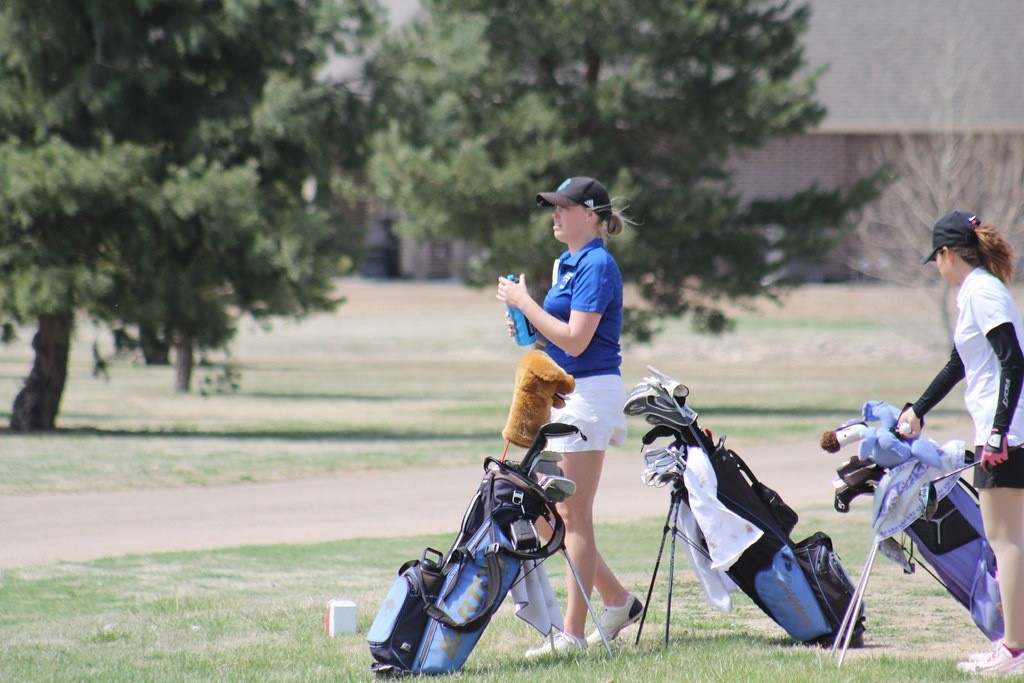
[898,422,912,436]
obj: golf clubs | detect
[821,422,890,513]
[626,376,701,489]
[518,421,585,500]
[920,441,1024,522]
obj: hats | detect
[922,211,981,264]
[536,177,612,219]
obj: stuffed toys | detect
[504,349,577,450]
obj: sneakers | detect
[957,644,1024,677]
[586,594,643,645]
[526,633,587,658]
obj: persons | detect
[897,209,1024,678]
[496,176,647,665]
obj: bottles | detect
[502,274,537,347]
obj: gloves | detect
[980,428,1009,475]
